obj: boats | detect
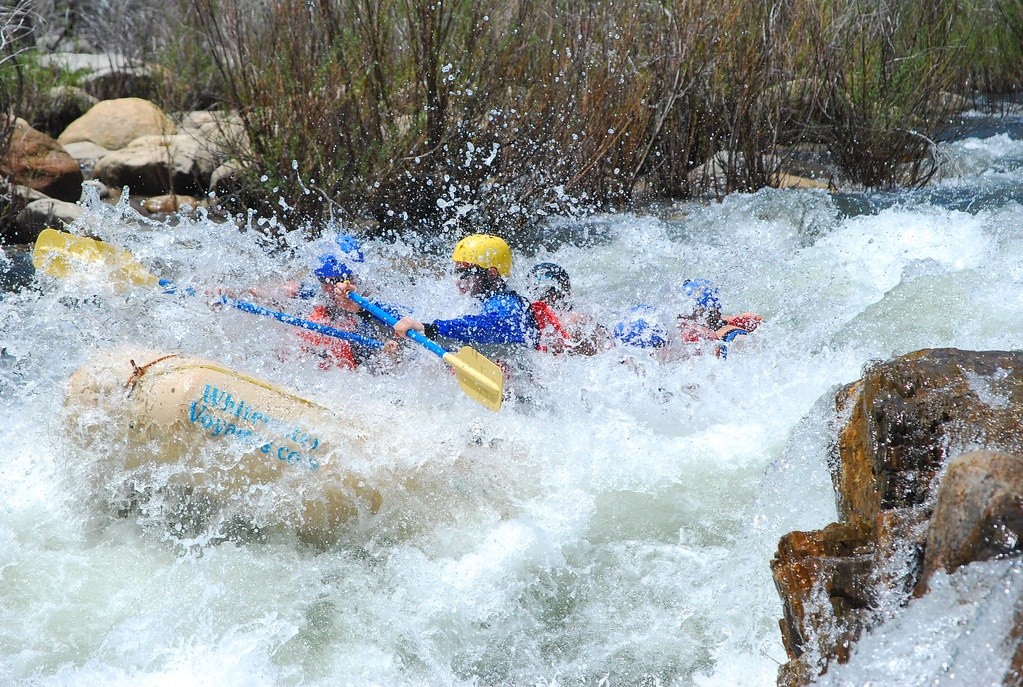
[62,341,520,554]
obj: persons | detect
[611,303,671,347]
[525,263,614,357]
[209,235,410,371]
[335,231,540,348]
[677,277,763,357]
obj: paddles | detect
[30,227,385,354]
[344,280,503,413]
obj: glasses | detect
[318,276,345,286]
[454,266,480,280]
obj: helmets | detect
[314,236,364,275]
[682,278,721,309]
[526,263,570,291]
[453,235,511,278]
[613,304,669,348]
